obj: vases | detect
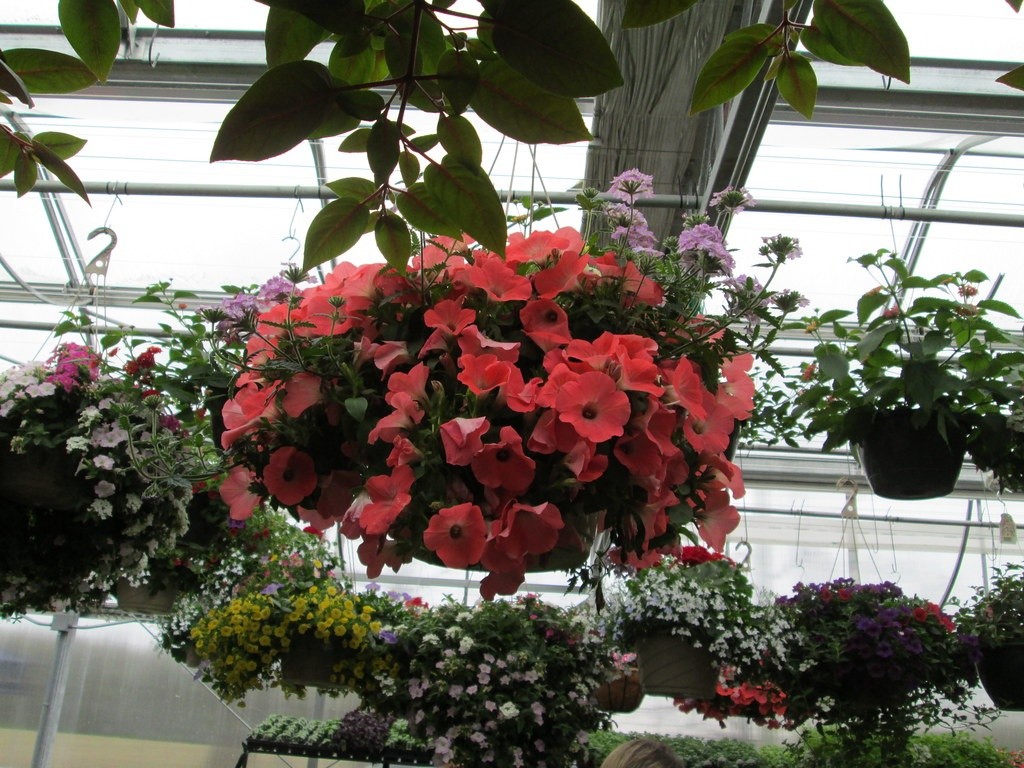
[624,621,722,700]
[395,512,600,571]
[862,413,966,500]
[282,640,347,688]
[976,646,1024,711]
[591,669,643,712]
[118,571,180,614]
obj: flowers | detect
[0,167,1024,768]
